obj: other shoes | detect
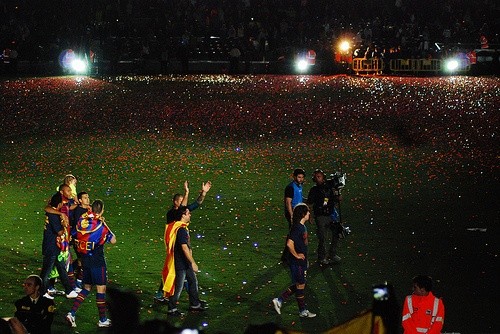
[43,293,55,300]
[66,290,78,298]
[154,297,169,304]
[319,259,330,265]
[48,287,65,295]
[74,287,82,292]
[189,305,207,312]
[168,310,185,317]
[330,255,341,262]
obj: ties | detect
[32,300,36,305]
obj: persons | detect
[38,175,91,300]
[279,171,311,268]
[402,273,445,334]
[0,317,31,334]
[272,203,317,319]
[166,206,208,315]
[155,180,211,302]
[13,275,56,334]
[66,200,116,327]
[0,0,500,76]
[307,169,342,264]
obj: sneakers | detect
[299,310,317,318]
[272,298,282,314]
[64,312,76,327]
[97,318,111,327]
